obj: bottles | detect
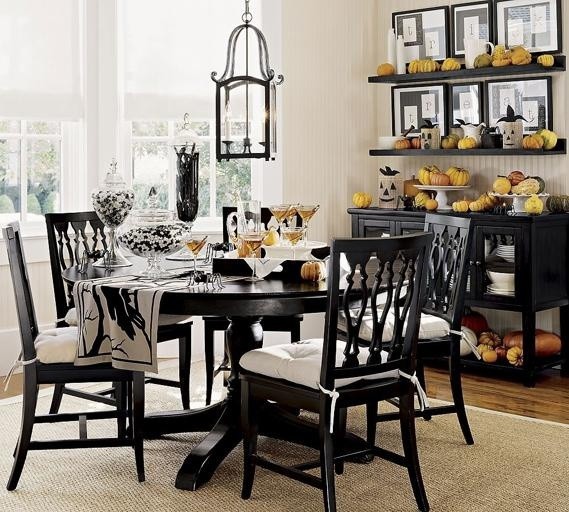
[386,27,406,75]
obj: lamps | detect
[211,0,284,163]
[165,112,206,260]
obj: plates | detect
[485,244,516,296]
[446,272,470,292]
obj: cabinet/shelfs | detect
[368,55,567,156]
[347,206,569,390]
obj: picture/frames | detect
[390,83,448,140]
[391,5,449,64]
[496,0,562,56]
[483,76,550,137]
[448,81,482,129]
[451,0,495,56]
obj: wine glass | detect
[180,231,208,283]
[119,239,185,278]
[238,229,268,283]
[412,183,473,213]
[268,200,318,245]
[280,227,306,259]
[91,208,134,268]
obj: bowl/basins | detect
[378,136,406,150]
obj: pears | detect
[263,230,279,245]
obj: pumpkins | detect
[404,166,569,214]
[395,128,557,151]
[458,311,562,366]
[353,191,372,208]
[301,261,326,283]
[377,44,555,77]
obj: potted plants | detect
[419,117,442,149]
[376,166,405,208]
[493,106,528,149]
[455,117,483,147]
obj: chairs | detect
[238,232,437,512]
[44,207,474,488]
[4,220,147,491]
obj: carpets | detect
[0,359,568,512]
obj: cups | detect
[227,200,263,240]
[462,36,496,70]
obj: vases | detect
[396,33,407,74]
[387,27,396,73]
